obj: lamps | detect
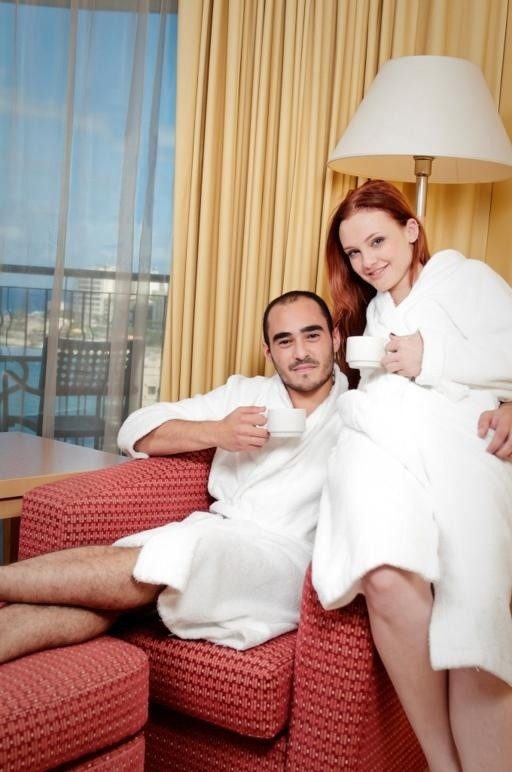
[327,54,512,226]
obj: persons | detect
[323,179,512,771]
[0,291,512,666]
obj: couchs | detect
[17,444,429,770]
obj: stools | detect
[1,635,150,771]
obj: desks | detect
[0,343,44,433]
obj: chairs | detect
[8,334,134,450]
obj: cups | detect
[255,407,309,439]
[345,335,392,370]
